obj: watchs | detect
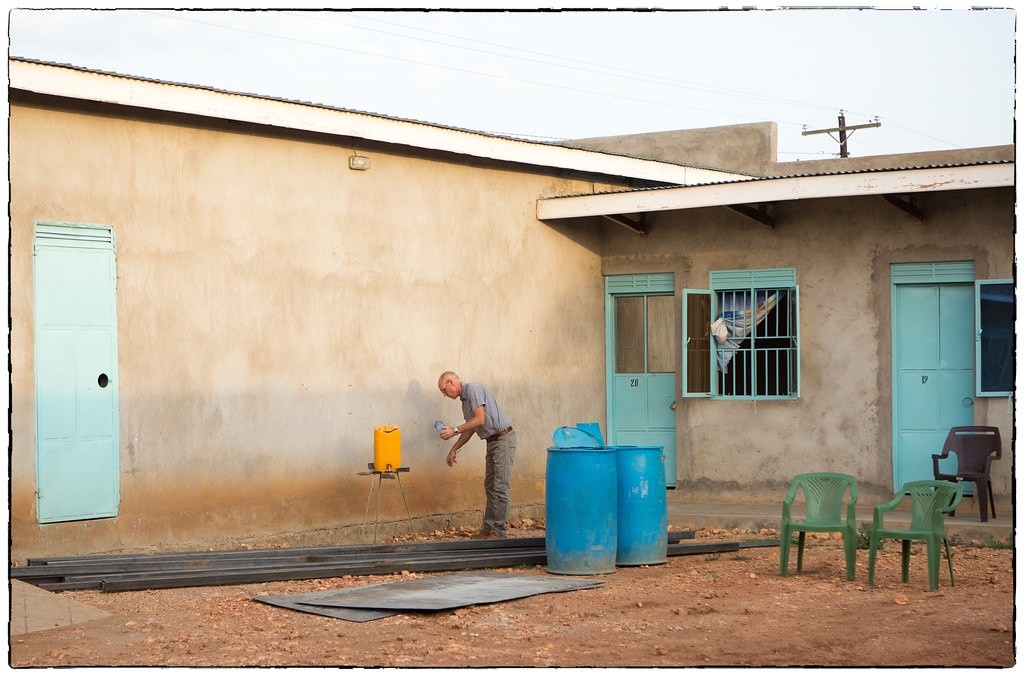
[454,427,459,435]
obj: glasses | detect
[441,383,448,393]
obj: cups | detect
[434,421,444,433]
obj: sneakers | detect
[469,529,490,539]
[485,531,505,539]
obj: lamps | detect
[349,145,371,171]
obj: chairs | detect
[869,480,964,592]
[779,472,858,580]
[932,426,1002,522]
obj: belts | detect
[486,426,513,442]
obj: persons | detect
[438,371,516,539]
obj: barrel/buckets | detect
[613,444,667,565]
[549,447,617,574]
